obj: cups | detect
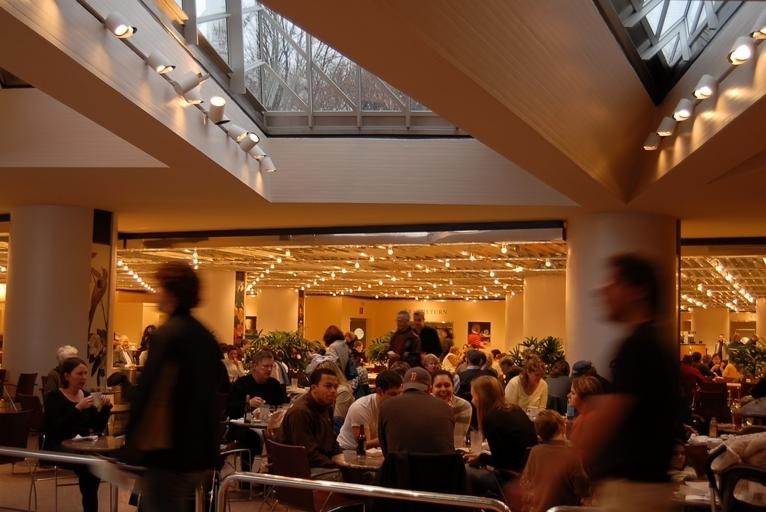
[526,406,538,417]
[305,386,310,391]
[470,431,482,451]
[731,414,742,430]
[342,449,356,463]
[291,378,298,389]
[90,391,102,408]
[259,404,271,426]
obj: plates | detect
[253,419,261,423]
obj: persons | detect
[122,261,231,510]
[539,252,684,510]
[224,310,621,512]
[682,328,766,435]
[42,322,156,512]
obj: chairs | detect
[1,350,766,512]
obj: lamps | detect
[78,1,278,174]
[642,29,766,151]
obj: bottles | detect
[710,417,718,437]
[356,422,367,455]
[243,393,252,423]
[567,400,575,419]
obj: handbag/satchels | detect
[345,352,359,381]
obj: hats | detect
[401,367,432,393]
